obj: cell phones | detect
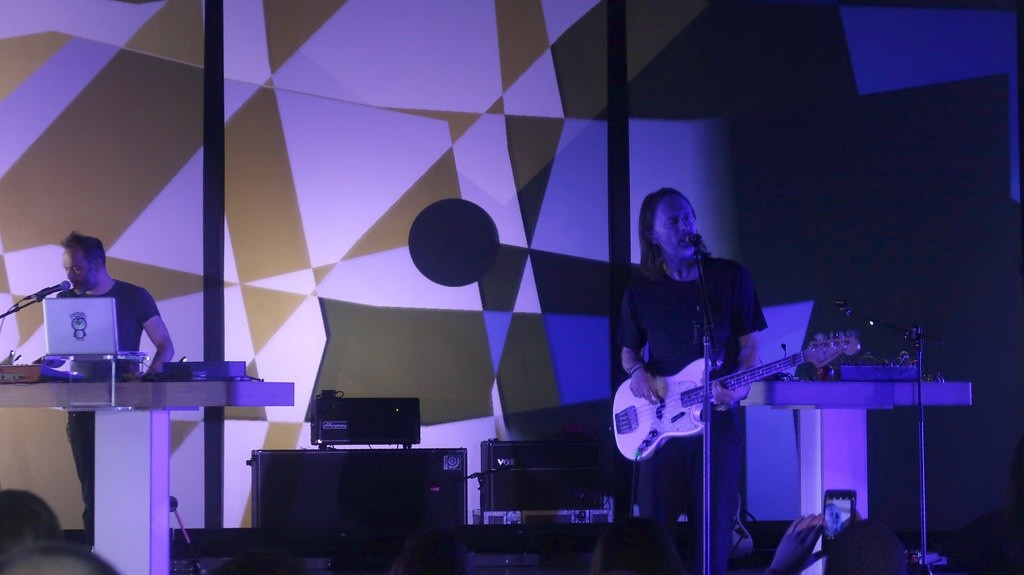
[821,489,857,554]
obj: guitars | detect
[612,328,863,463]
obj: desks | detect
[0,382,295,575]
[745,380,974,575]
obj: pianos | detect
[0,380,294,408]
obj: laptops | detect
[43,297,147,357]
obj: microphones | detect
[25,281,71,300]
[685,234,702,247]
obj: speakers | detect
[480,440,604,514]
[251,449,468,541]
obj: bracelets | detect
[628,363,641,375]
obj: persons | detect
[591,516,687,575]
[620,186,768,575]
[388,528,477,575]
[0,490,120,575]
[826,506,850,539]
[37,233,173,546]
[766,515,906,574]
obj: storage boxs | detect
[480,437,603,512]
[248,447,468,552]
